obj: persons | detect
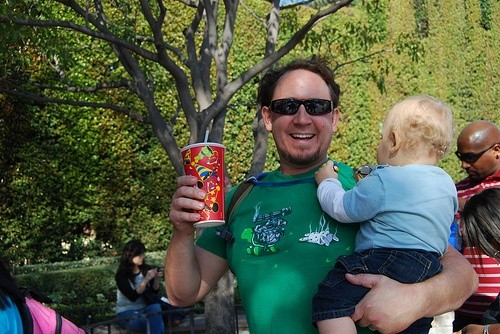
[164,60,500,334]
[115,239,165,334]
[0,255,89,334]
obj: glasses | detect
[454,143,500,162]
[268,98,333,115]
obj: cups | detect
[180,142,227,228]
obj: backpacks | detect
[17,288,87,334]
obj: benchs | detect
[75,313,248,334]
